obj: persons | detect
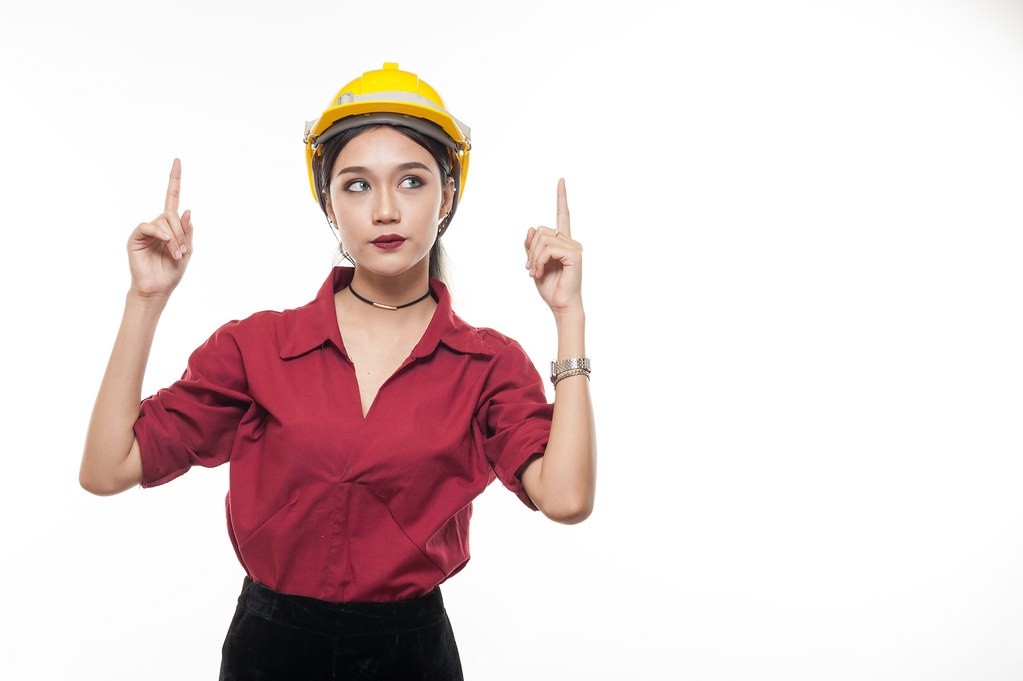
[78,62,598,681]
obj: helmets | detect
[304,62,472,237]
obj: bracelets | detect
[549,357,591,388]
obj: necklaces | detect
[349,285,431,311]
